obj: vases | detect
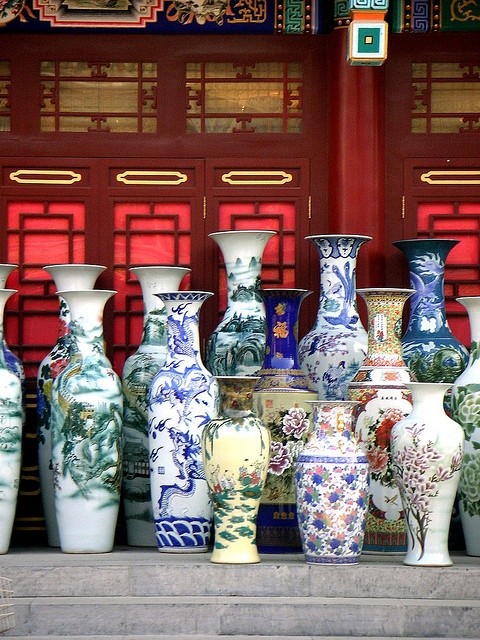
[154,292,217,554]
[202,377,265,564]
[299,234,369,401]
[292,402,368,565]
[0,264,24,382]
[39,264,109,546]
[208,231,275,378]
[249,289,316,554]
[445,298,480,560]
[52,290,123,558]
[0,288,26,557]
[391,383,463,566]
[348,288,414,554]
[121,266,190,547]
[392,240,472,417]
